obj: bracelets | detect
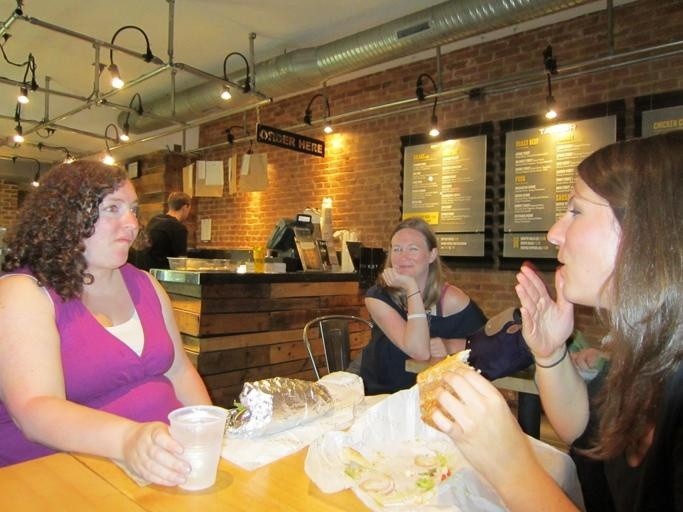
[530,343,568,368]
[406,290,420,299]
[406,313,426,319]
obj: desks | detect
[401,348,541,442]
[0,413,560,510]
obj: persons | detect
[429,128,683,512]
[144,190,190,261]
[125,203,169,270]
[0,158,219,488]
[355,215,481,393]
[567,328,610,380]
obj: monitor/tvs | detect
[267,218,314,249]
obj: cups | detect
[252,244,266,274]
[166,404,228,490]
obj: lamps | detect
[0,24,559,162]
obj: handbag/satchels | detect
[466,305,578,383]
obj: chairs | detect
[298,314,375,380]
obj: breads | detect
[239,378,331,429]
[417,348,481,432]
